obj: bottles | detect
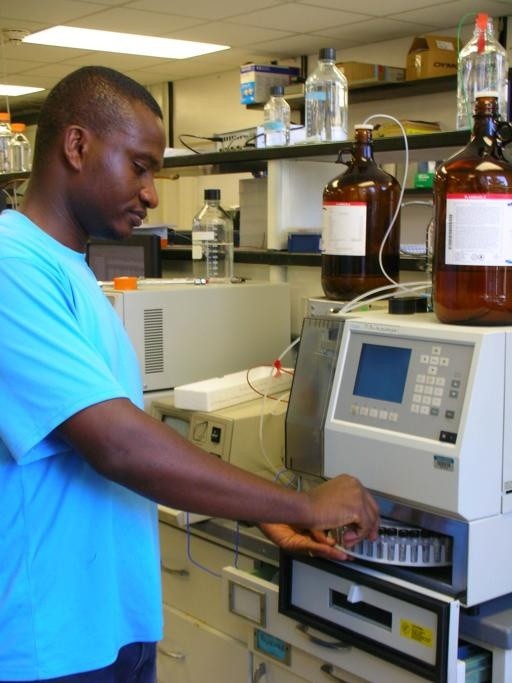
[431,91,512,326]
[191,188,234,283]
[320,124,402,300]
[455,16,509,131]
[0,111,32,175]
[304,47,350,144]
[263,85,291,148]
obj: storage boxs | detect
[239,62,299,105]
[335,62,406,83]
[406,35,463,81]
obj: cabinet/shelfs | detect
[154,65,512,272]
[155,514,512,683]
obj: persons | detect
[1,64,380,682]
